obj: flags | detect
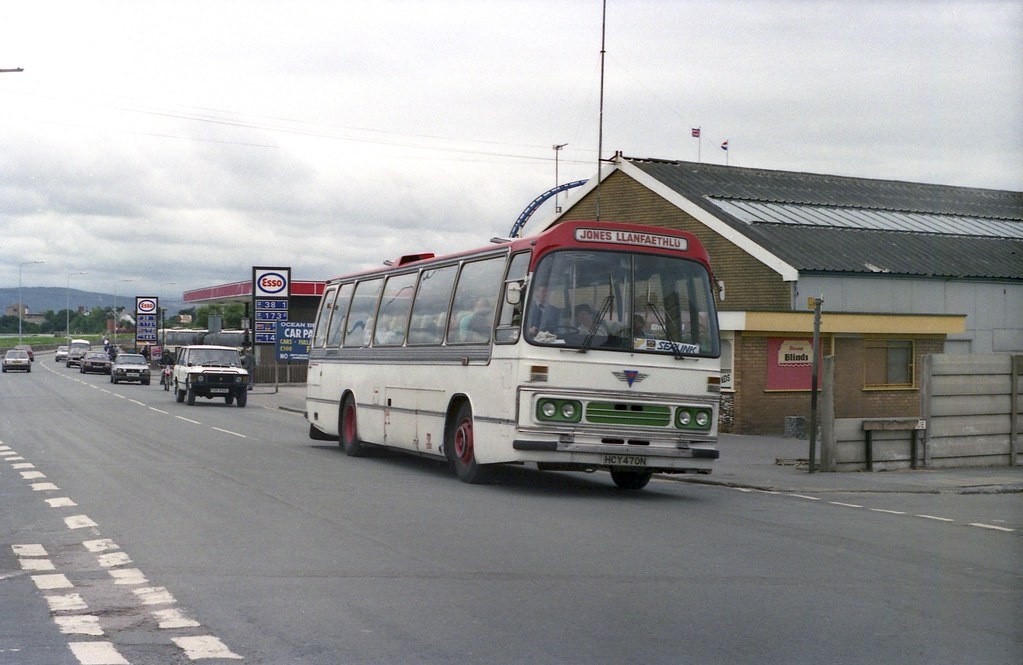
[692,128,700,137]
[721,141,727,150]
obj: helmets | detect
[164,349,170,353]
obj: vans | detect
[69,339,91,352]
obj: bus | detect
[305,221,726,492]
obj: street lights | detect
[160,281,177,306]
[114,279,134,345]
[554,142,569,209]
[17,260,46,345]
[66,270,90,345]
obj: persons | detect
[104,343,115,354]
[104,339,109,344]
[576,305,607,336]
[459,298,494,342]
[142,346,148,355]
[189,351,201,364]
[158,349,175,386]
[634,315,655,338]
[529,285,560,334]
[245,349,256,390]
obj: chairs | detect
[364,310,471,344]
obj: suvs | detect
[172,345,250,408]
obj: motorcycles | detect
[159,363,176,391]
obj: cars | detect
[67,349,87,367]
[111,353,150,385]
[81,351,111,375]
[15,343,35,361]
[1,350,32,374]
[55,345,68,362]
